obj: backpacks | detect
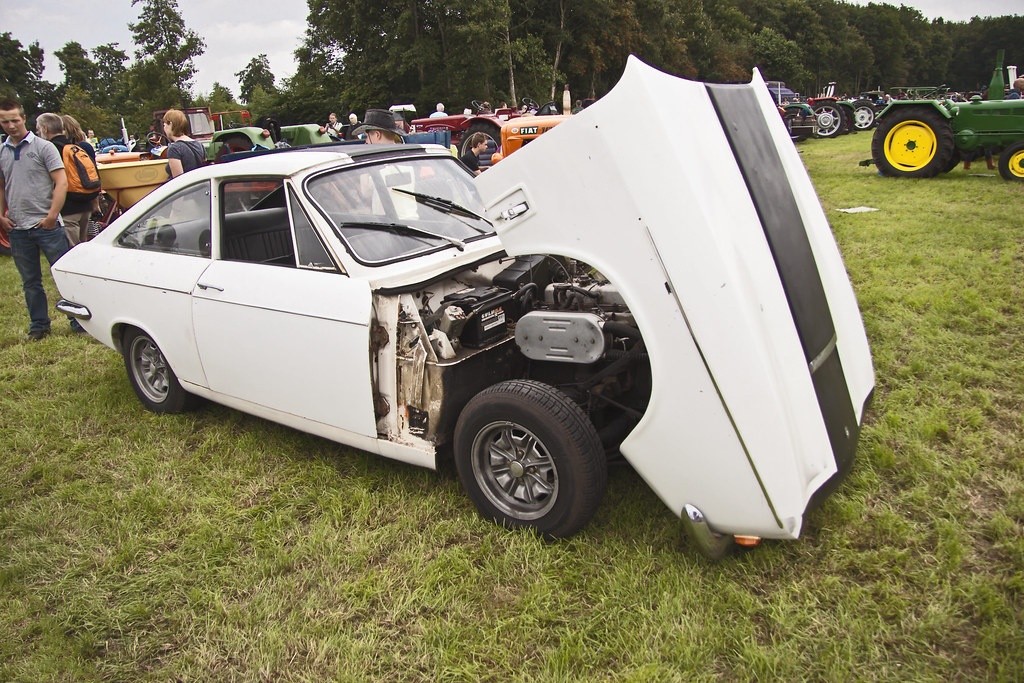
[176,139,215,167]
[53,140,101,194]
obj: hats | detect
[350,108,408,137]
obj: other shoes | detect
[989,165,997,170]
[73,326,86,332]
[28,329,51,341]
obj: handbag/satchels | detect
[88,213,99,237]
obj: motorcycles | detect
[90,102,572,235]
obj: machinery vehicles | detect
[766,45,1024,183]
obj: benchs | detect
[126,207,333,268]
[346,227,417,261]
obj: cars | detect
[50,53,876,564]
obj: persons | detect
[149,224,177,251]
[352,108,420,220]
[324,112,343,137]
[572,100,584,114]
[162,109,207,219]
[128,122,159,151]
[36,112,101,246]
[465,101,537,115]
[1006,78,1024,99]
[345,114,362,140]
[459,132,490,175]
[88,128,98,146]
[429,103,448,118]
[0,97,85,340]
